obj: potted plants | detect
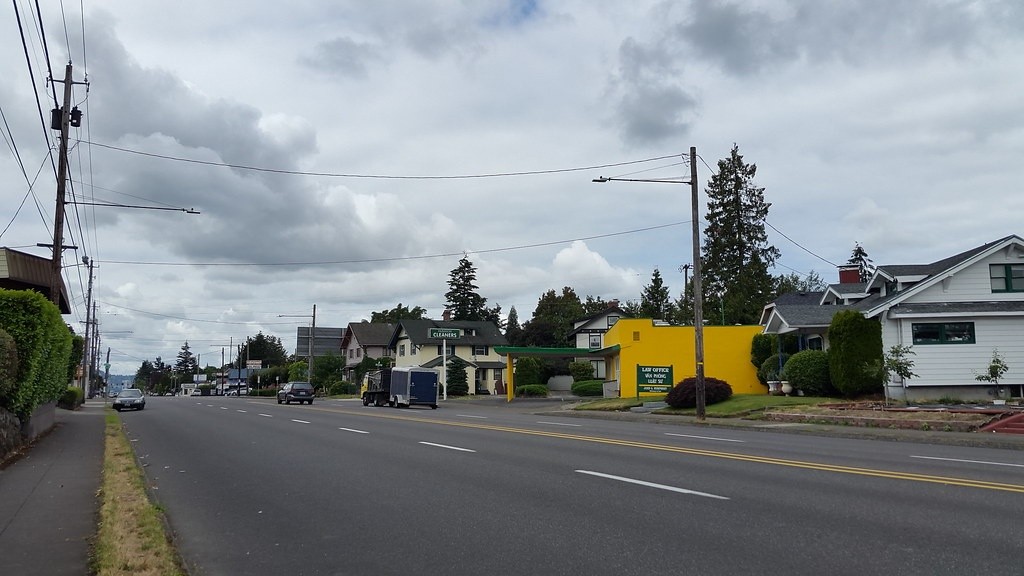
[766,371,780,395]
[780,372,793,396]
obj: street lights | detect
[592,146,707,423]
[105,346,111,398]
[276,304,316,385]
[48,202,202,310]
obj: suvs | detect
[277,381,315,404]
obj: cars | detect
[224,389,236,396]
[113,389,146,412]
[231,387,252,396]
[190,389,201,396]
[114,392,119,397]
[150,392,154,396]
[108,393,114,398]
[94,391,102,398]
[153,393,159,396]
[165,392,172,397]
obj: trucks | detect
[360,367,391,407]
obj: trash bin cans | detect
[603,379,618,398]
